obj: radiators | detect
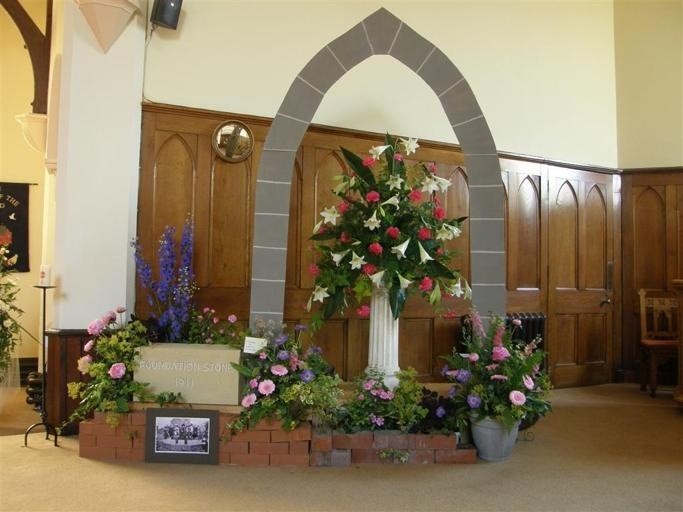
[462,310,545,369]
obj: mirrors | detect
[212,120,254,163]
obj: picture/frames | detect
[145,409,219,465]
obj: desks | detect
[673,279,683,407]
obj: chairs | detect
[638,288,679,397]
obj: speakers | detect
[150,0,182,30]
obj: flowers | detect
[438,305,548,418]
[354,368,428,425]
[196,307,241,343]
[305,133,469,316]
[66,305,145,397]
[130,211,197,336]
[240,318,349,420]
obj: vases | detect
[468,414,522,462]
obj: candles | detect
[39,265,50,286]
